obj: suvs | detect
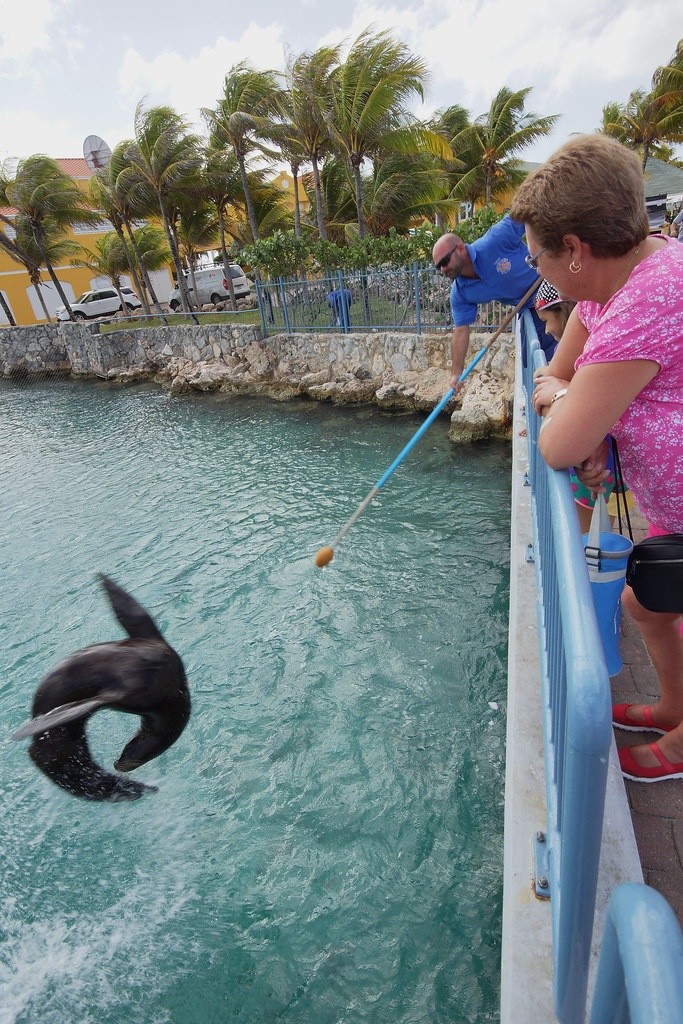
[55,284,143,322]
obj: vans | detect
[168,261,250,313]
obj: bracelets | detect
[551,388,568,403]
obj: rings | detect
[535,393,537,398]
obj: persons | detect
[510,133,683,783]
[669,209,683,243]
[327,290,352,333]
[534,277,632,536]
[432,214,567,397]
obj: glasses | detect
[525,248,548,270]
[435,245,458,271]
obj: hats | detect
[534,278,564,311]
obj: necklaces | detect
[614,237,648,294]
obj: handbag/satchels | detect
[577,481,633,677]
[626,533,683,614]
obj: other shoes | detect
[618,742,683,784]
[612,703,675,735]
